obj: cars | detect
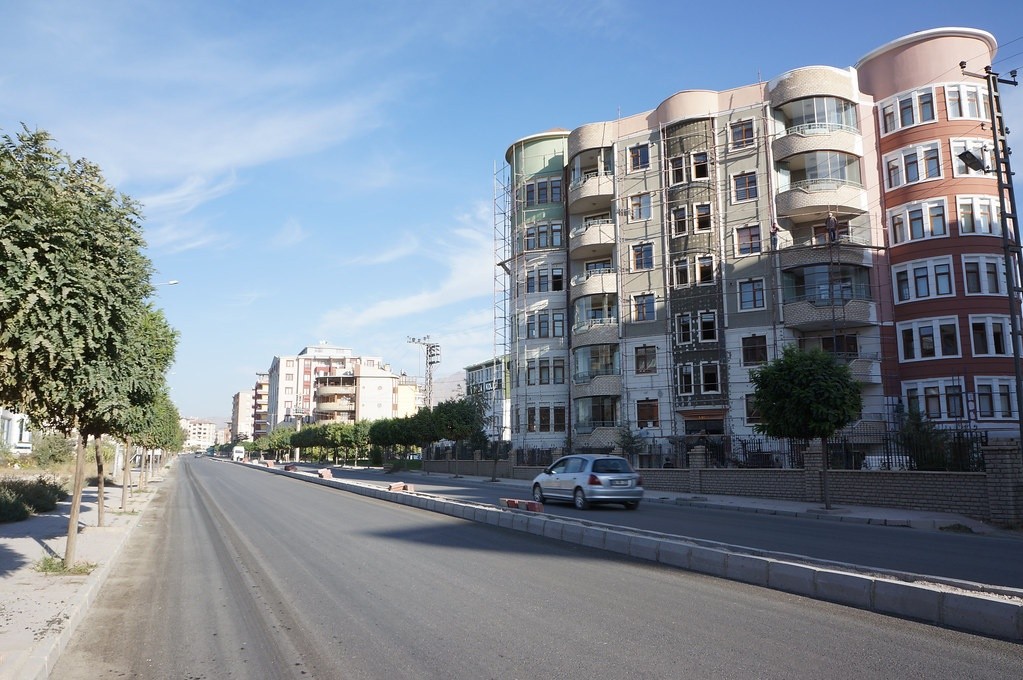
[531,453,645,510]
[194,451,203,458]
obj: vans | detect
[231,445,245,461]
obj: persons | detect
[825,213,837,245]
[769,223,782,252]
